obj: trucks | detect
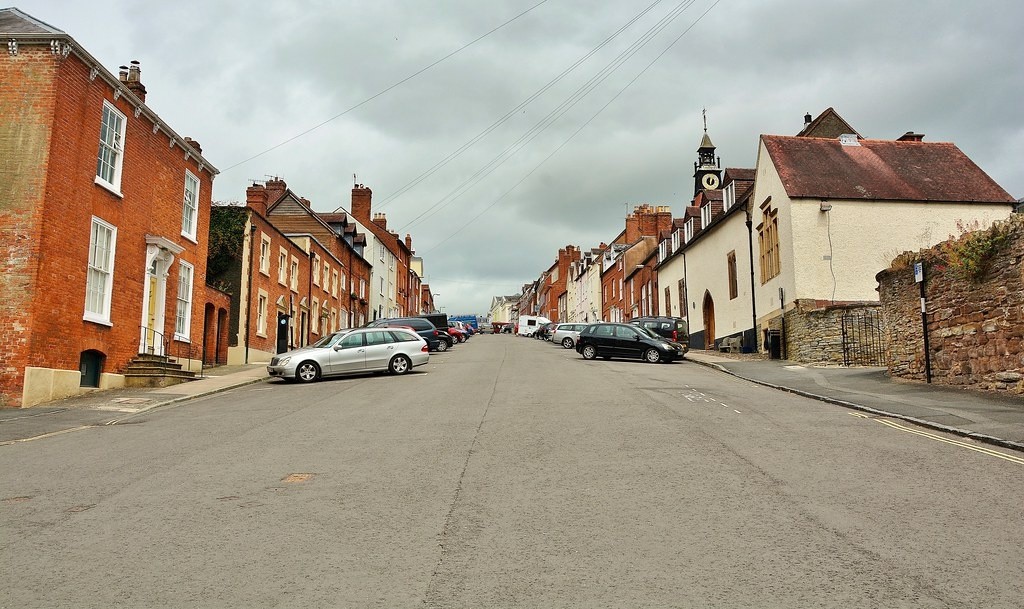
[476,316,494,335]
[518,315,552,337]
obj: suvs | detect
[553,323,608,349]
[418,314,450,333]
[351,317,440,353]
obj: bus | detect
[492,322,513,334]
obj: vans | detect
[617,316,689,354]
[450,316,479,332]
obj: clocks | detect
[698,171,721,190]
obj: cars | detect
[436,330,453,352]
[536,322,568,343]
[447,319,475,344]
[576,322,684,364]
[266,323,429,384]
